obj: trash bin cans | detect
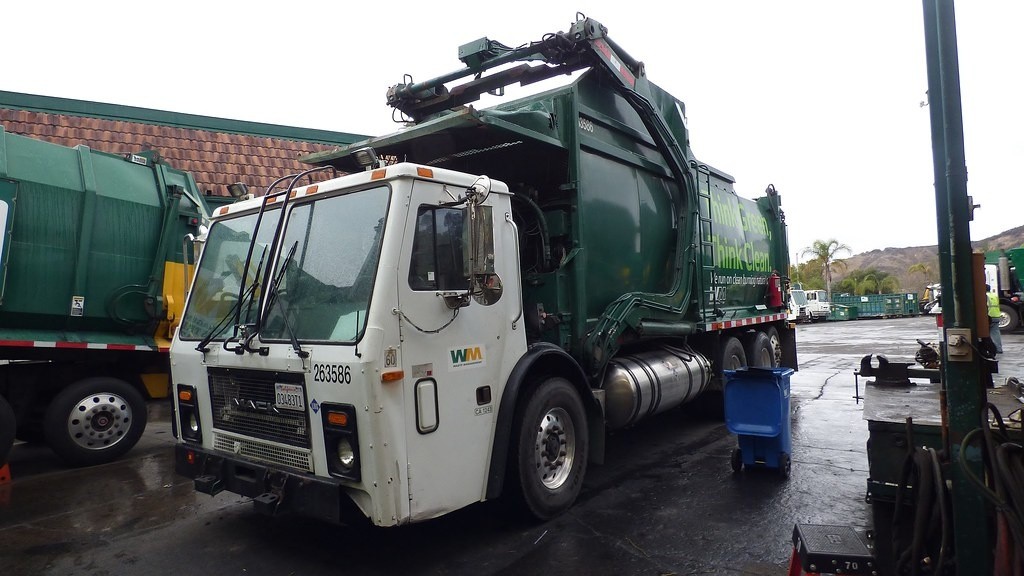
[721,364,796,483]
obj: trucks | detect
[788,289,809,325]
[805,290,832,323]
[986,248,1024,332]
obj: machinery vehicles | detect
[167,8,798,536]
[0,127,210,458]
